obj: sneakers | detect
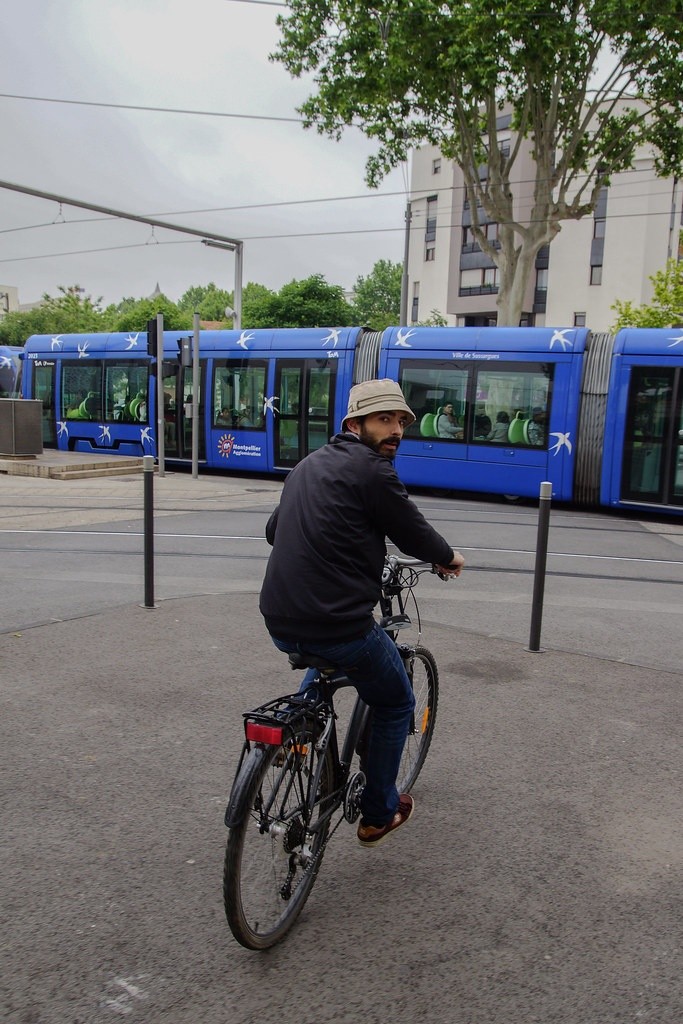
[357,793,414,847]
[267,747,309,772]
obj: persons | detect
[486,411,511,443]
[64,388,87,413]
[164,392,194,449]
[630,394,655,492]
[220,406,266,428]
[528,407,546,445]
[258,378,464,848]
[510,409,524,423]
[118,395,136,421]
[436,402,465,439]
[292,401,299,413]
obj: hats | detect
[341,379,416,431]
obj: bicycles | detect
[222,555,463,951]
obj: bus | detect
[0,347,22,399]
[13,325,683,521]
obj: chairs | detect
[78,391,239,429]
[503,409,533,446]
[418,406,465,439]
